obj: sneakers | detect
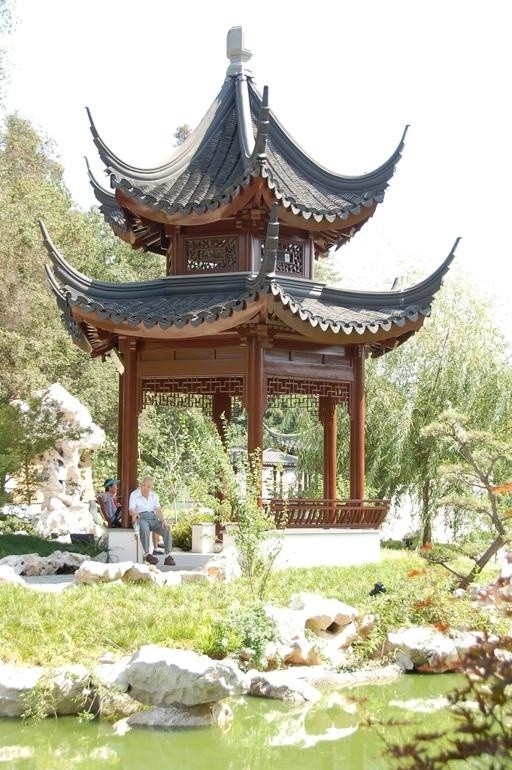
[153,547,165,555]
[158,540,164,548]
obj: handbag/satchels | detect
[110,506,122,528]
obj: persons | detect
[127,476,177,565]
[151,530,165,556]
[99,474,165,559]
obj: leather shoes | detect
[145,553,159,564]
[164,555,176,565]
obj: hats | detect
[104,479,120,488]
